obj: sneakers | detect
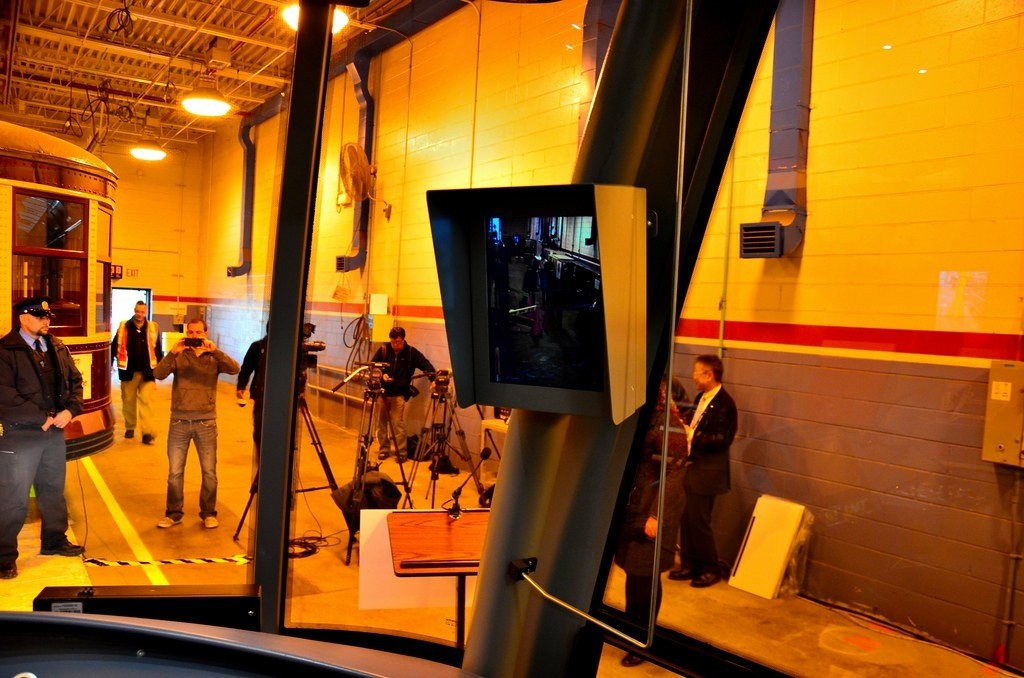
[205,514,218,528]
[158,517,182,528]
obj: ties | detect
[34,339,42,352]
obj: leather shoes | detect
[692,571,720,586]
[670,567,696,579]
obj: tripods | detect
[232,385,501,566]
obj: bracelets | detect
[651,515,657,520]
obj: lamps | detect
[130,135,166,161]
[181,74,230,116]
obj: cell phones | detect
[184,338,202,346]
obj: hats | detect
[13,296,55,318]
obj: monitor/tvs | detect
[425,185,612,420]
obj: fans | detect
[340,142,387,205]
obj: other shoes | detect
[0,563,16,578]
[378,452,388,459]
[40,540,84,556]
[622,652,643,667]
[125,429,134,438]
[143,434,154,442]
[396,455,406,464]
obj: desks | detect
[387,510,490,668]
[480,419,508,490]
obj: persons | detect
[370,327,437,463]
[0,297,85,580]
[614,378,688,667]
[670,376,692,420]
[153,318,241,528]
[668,353,738,587]
[238,320,307,460]
[111,301,164,443]
[523,246,554,272]
